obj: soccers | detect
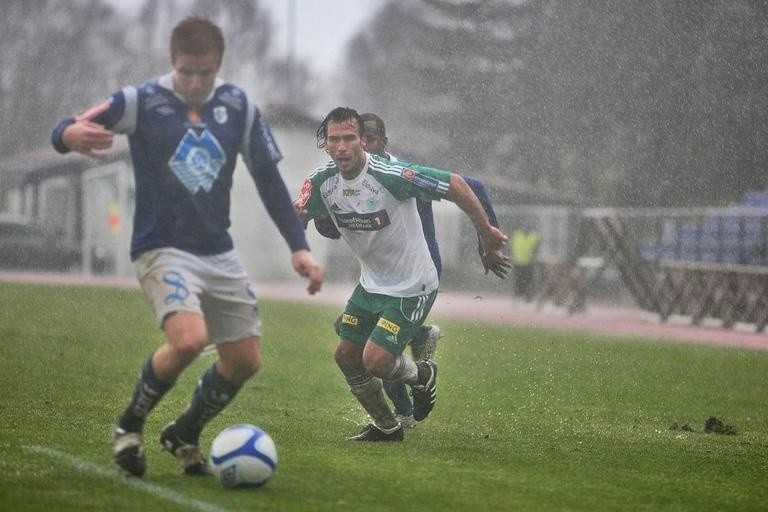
[210,423,279,487]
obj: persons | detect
[292,106,509,442]
[51,14,324,479]
[313,111,512,431]
[510,218,538,297]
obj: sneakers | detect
[347,325,440,441]
[157,420,220,476]
[114,409,146,478]
